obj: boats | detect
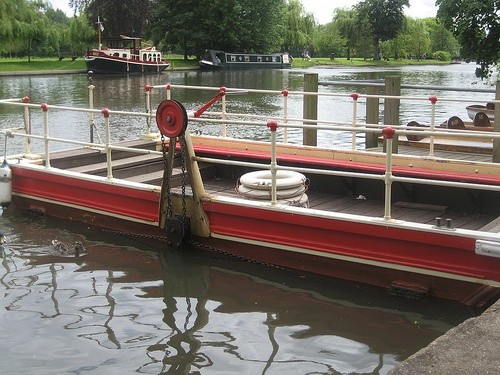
[86,36,170,73]
[199,49,292,69]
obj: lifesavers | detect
[240,170,306,190]
[244,193,308,205]
[297,203,307,208]
[238,184,306,199]
